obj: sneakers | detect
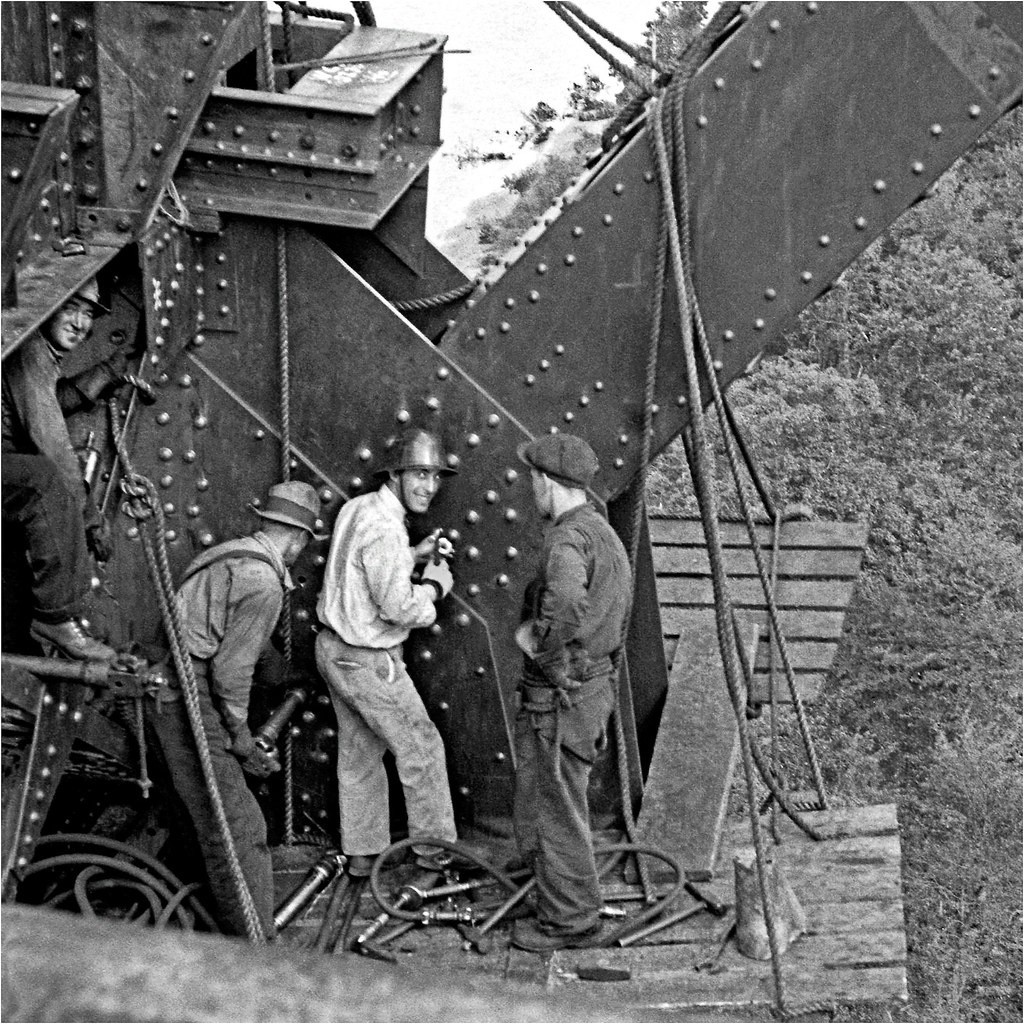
[507,916,604,956]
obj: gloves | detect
[229,724,258,757]
[77,350,127,402]
[422,557,455,600]
[514,622,580,708]
[79,480,117,562]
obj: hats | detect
[62,273,107,311]
[247,479,330,540]
[518,432,601,484]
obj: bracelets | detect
[421,577,444,600]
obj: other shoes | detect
[420,842,494,870]
[349,840,405,881]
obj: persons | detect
[3,277,116,661]
[314,430,491,876]
[504,432,635,952]
[150,482,318,948]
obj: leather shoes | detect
[29,613,116,664]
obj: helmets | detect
[370,427,455,471]
